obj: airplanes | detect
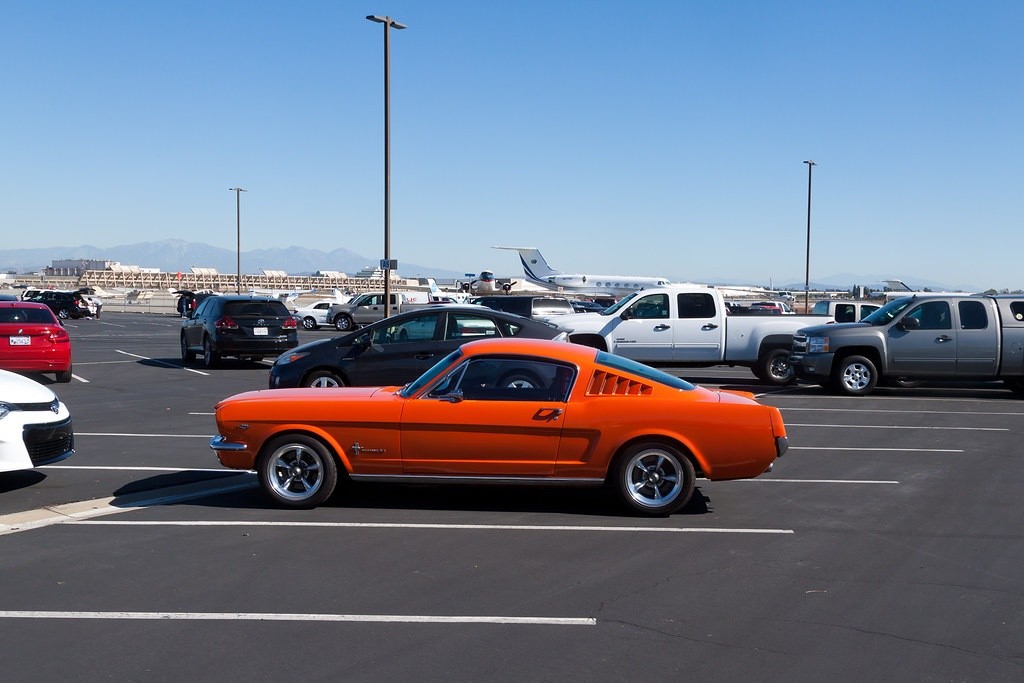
[492,245,674,297]
[454,270,518,295]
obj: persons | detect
[180,295,192,319]
[88,297,102,320]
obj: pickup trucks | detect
[790,293,1024,397]
[553,286,835,388]
[328,291,453,332]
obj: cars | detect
[0,284,102,321]
[0,364,77,473]
[291,298,344,329]
[263,304,577,388]
[717,287,885,325]
[207,336,792,518]
[173,288,219,317]
[0,301,73,383]
[178,296,299,365]
[436,290,622,318]
